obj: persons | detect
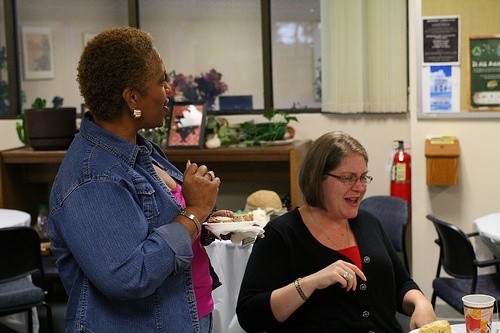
[236,132,437,333]
[48,27,265,333]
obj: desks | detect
[201,239,254,332]
[0,208,39,333]
[473,214,500,275]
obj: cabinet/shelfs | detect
[0,141,312,254]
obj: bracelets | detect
[295,278,308,301]
[179,211,201,232]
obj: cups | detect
[462,294,495,333]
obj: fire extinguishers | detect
[390,140,411,225]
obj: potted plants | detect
[238,108,298,149]
[24,97,78,151]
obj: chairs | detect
[360,196,411,276]
[425,214,500,324]
[0,226,54,333]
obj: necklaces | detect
[309,207,350,253]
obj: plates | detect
[202,220,259,229]
[258,139,295,146]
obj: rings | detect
[344,272,349,278]
[207,172,213,181]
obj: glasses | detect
[325,173,373,185]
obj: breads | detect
[417,319,452,333]
[232,213,254,222]
[247,190,283,209]
[207,217,234,223]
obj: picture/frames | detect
[165,100,208,150]
[20,24,56,81]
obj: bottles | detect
[37,205,49,236]
